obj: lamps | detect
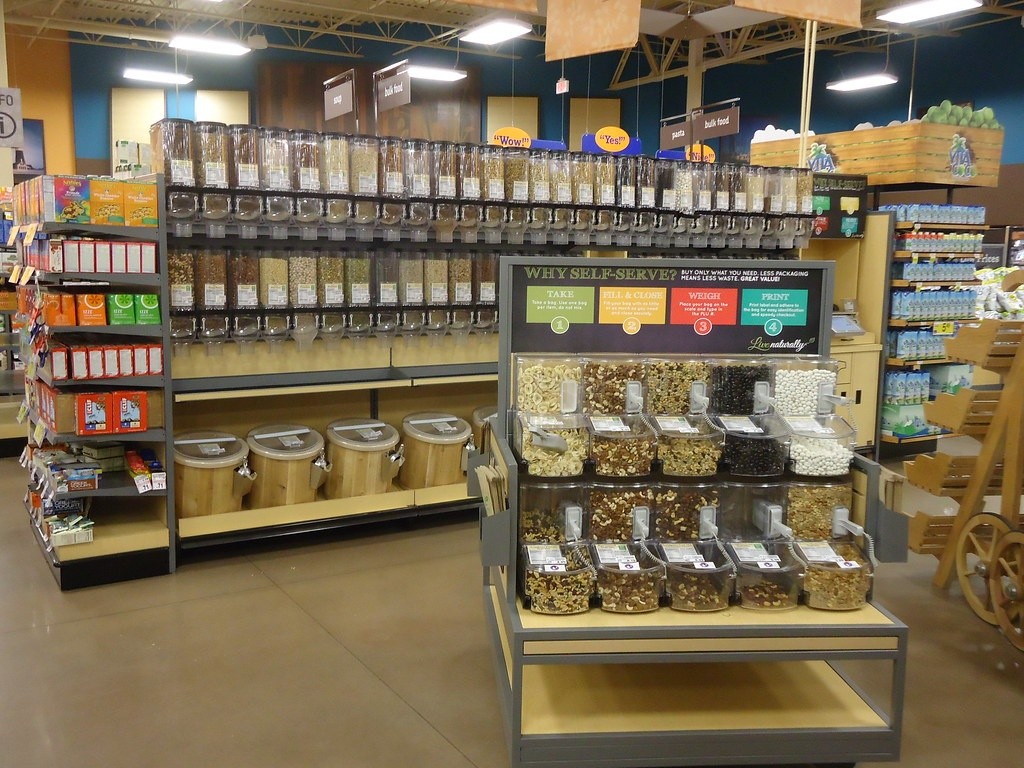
[826,48,900,92]
[457,10,533,45]
[874,0,984,26]
[397,36,468,82]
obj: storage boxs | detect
[512,356,875,615]
[13,174,161,546]
[812,175,869,238]
[751,122,1004,192]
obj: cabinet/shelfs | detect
[484,255,911,768]
[878,222,1024,444]
[0,245,30,460]
[167,184,814,551]
[904,316,1024,649]
[829,344,883,451]
[11,174,177,593]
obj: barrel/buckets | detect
[325,418,405,499]
[247,424,331,509]
[173,431,256,516]
[400,413,476,490]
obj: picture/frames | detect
[12,118,46,176]
